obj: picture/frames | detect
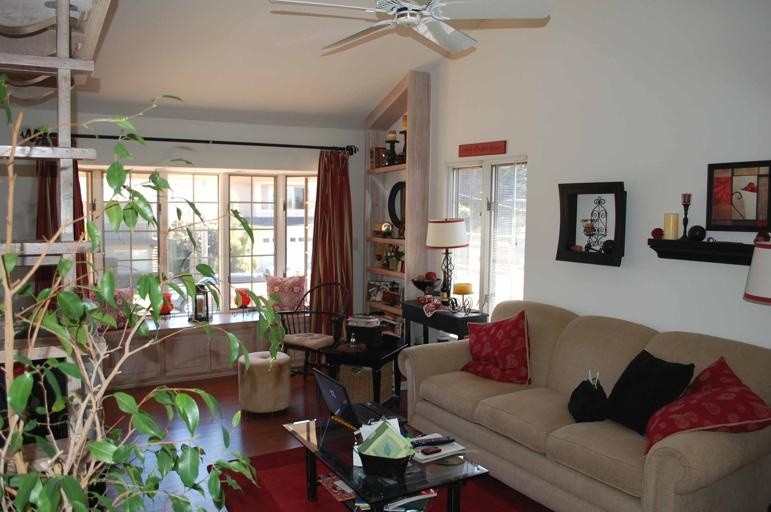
[705,158,771,233]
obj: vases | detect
[387,257,397,270]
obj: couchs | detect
[397,300,770,512]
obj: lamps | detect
[187,284,213,323]
[425,216,469,305]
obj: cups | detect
[662,212,681,241]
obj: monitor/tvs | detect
[1,301,103,462]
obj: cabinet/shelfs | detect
[362,72,430,349]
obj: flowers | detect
[383,245,404,260]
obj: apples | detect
[426,272,436,280]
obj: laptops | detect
[311,367,408,430]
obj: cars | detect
[98,266,158,292]
[170,271,265,314]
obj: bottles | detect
[159,292,174,315]
[234,288,250,308]
[439,281,450,307]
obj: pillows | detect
[608,349,695,438]
[262,273,306,316]
[642,355,771,460]
[460,310,533,386]
[90,283,134,332]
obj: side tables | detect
[318,340,402,411]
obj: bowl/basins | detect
[411,278,441,297]
[357,441,415,476]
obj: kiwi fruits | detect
[417,274,425,282]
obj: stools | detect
[237,349,292,414]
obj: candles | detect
[682,193,690,203]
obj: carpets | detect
[207,445,549,512]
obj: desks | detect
[401,299,490,347]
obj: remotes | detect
[413,436,455,446]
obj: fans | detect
[270,0,551,55]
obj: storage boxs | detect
[340,360,393,406]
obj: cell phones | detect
[421,447,441,455]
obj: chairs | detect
[276,281,350,403]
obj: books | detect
[353,415,415,460]
[409,431,467,464]
